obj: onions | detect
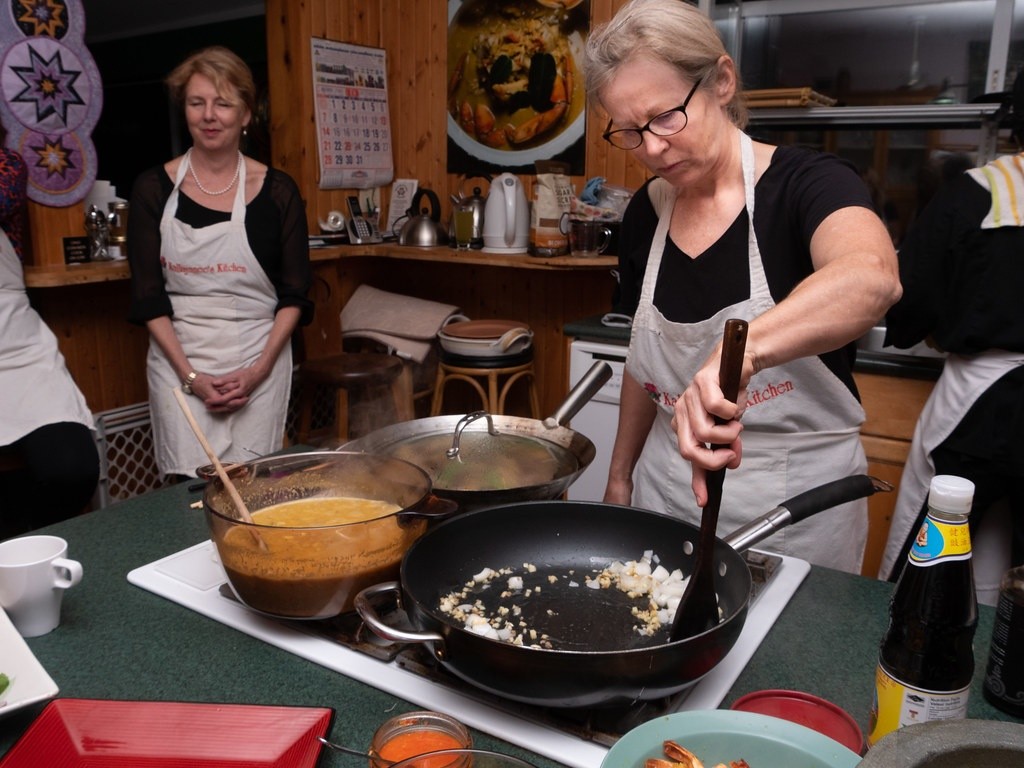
[456,549,693,642]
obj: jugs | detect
[450,171,493,250]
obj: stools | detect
[297,351,405,450]
[430,354,542,420]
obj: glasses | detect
[602,75,703,149]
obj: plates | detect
[599,709,863,768]
[0,606,60,715]
[448,1,585,166]
[442,320,530,338]
[0,698,336,768]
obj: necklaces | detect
[188,150,241,195]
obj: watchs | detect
[182,370,198,394]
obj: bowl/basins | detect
[869,327,916,355]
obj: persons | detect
[583,0,903,575]
[127,48,315,486]
[879,71,1024,608]
[0,229,101,543]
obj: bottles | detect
[107,236,127,259]
[859,474,980,756]
[368,709,474,768]
[982,564,1024,721]
[108,201,128,235]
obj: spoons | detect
[83,204,117,258]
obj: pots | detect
[187,454,458,622]
[432,314,534,368]
[352,360,613,532]
[353,474,894,708]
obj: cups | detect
[82,179,115,218]
[1,536,83,637]
[568,221,612,258]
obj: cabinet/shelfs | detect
[850,374,936,578]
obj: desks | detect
[23,238,619,289]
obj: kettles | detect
[482,172,530,253]
[392,188,448,247]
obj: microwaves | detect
[693,0,1024,109]
